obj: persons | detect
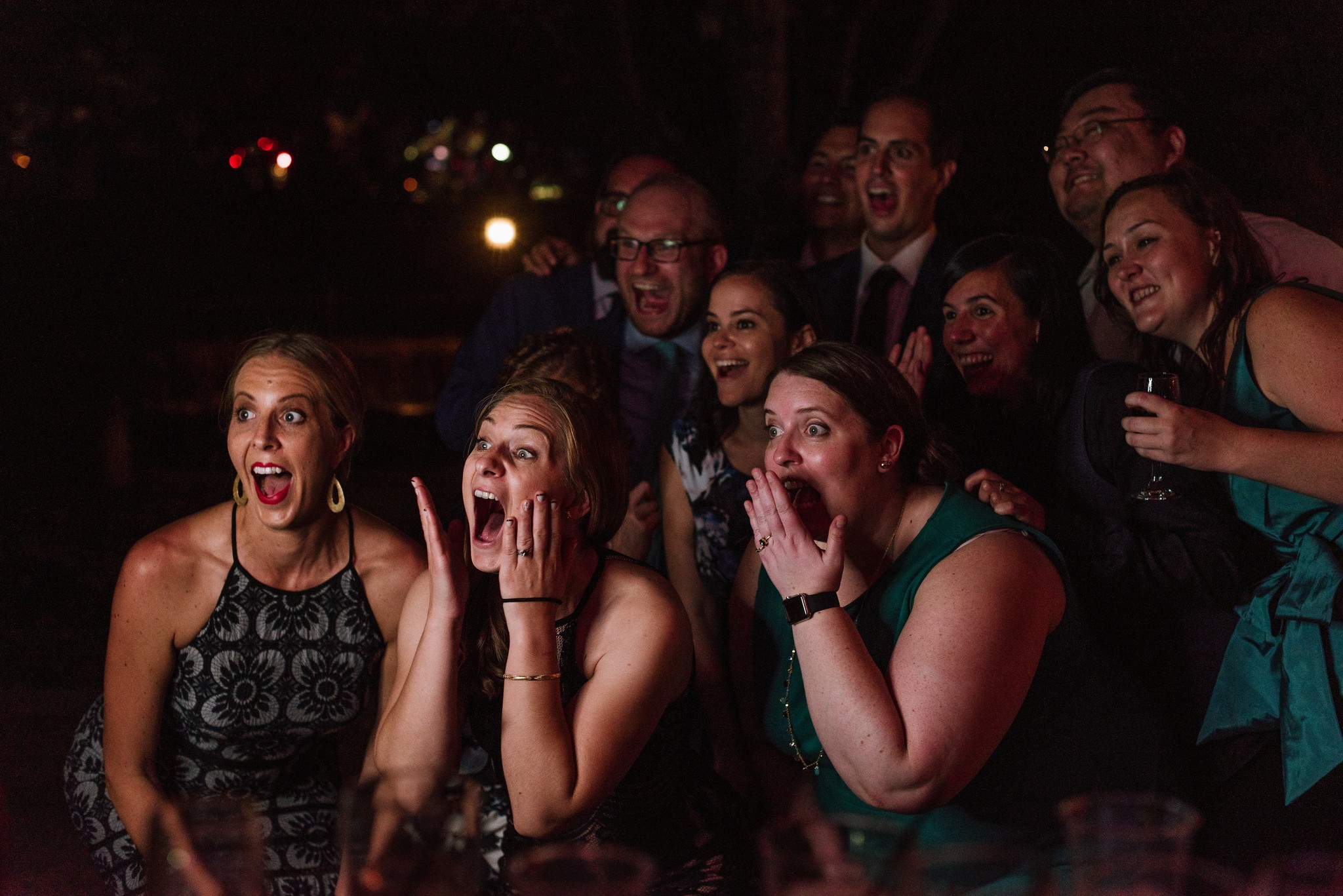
[891,233,1284,781]
[734,123,862,271]
[1086,169,1343,810]
[577,172,737,445]
[497,328,737,591]
[659,259,1046,764]
[1042,67,1341,362]
[376,377,708,836]
[438,155,676,449]
[774,87,1005,365]
[731,339,1074,817]
[64,330,425,794]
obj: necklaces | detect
[779,495,907,776]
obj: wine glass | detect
[1130,370,1186,502]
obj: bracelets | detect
[500,597,562,605]
[501,674,562,681]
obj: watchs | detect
[781,591,840,626]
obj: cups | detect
[1053,789,1203,895]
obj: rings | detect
[1000,483,1004,491]
[756,546,764,552]
[515,547,533,556]
[759,534,772,546]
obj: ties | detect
[1039,115,1151,164]
[858,266,901,357]
[649,340,684,466]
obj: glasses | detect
[599,190,631,218]
[607,235,713,263]
